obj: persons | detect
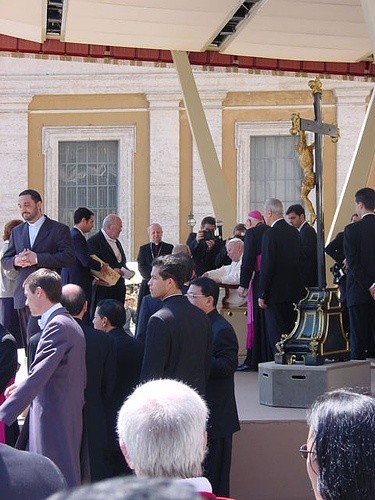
[61,208,111,325]
[236,210,271,372]
[0,189,76,358]
[135,223,174,337]
[256,198,301,358]
[344,188,375,361]
[136,243,241,498]
[0,322,21,447]
[92,298,144,406]
[0,268,87,487]
[0,219,25,349]
[215,224,247,268]
[186,217,232,279]
[199,238,249,314]
[325,213,360,338]
[115,379,242,500]
[286,204,318,297]
[0,442,71,500]
[299,389,375,500]
[27,283,110,480]
[86,214,135,319]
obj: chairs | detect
[217,283,248,356]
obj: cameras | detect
[203,228,212,241]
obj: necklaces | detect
[150,242,163,259]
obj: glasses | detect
[183,293,207,300]
[299,444,319,459]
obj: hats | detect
[247,210,263,220]
